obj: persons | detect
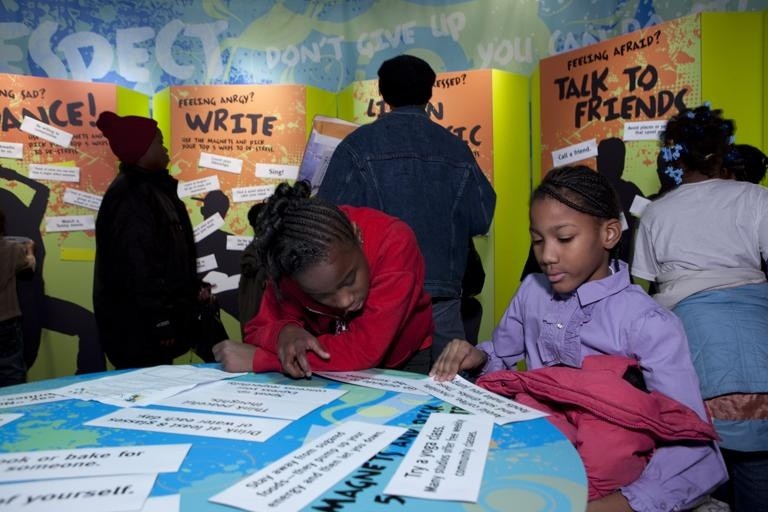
[430,165,729,511]
[629,104,768,511]
[89,110,217,369]
[216,179,435,385]
[318,54,499,390]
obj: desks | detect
[1,364,587,512]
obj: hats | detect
[96,111,158,164]
[378,55,436,107]
